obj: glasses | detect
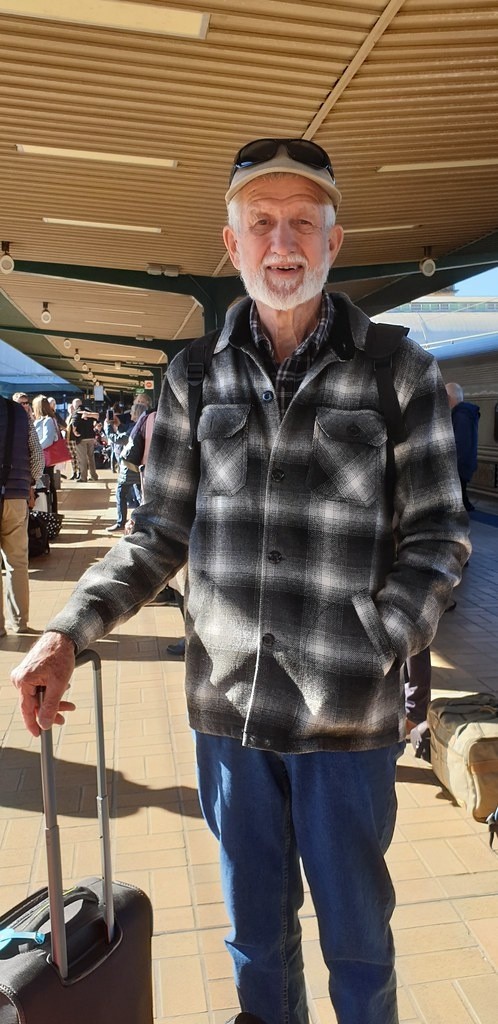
[20,402,30,406]
[228,138,334,187]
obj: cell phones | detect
[109,411,114,420]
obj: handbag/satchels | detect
[43,417,73,466]
[122,415,148,466]
[33,511,64,538]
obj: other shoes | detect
[166,645,185,655]
[106,524,125,532]
[0,628,6,637]
[77,479,87,482]
[89,478,98,481]
[5,620,29,633]
[71,474,77,480]
[76,474,80,479]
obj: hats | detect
[225,137,342,216]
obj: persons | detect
[0,392,188,656]
[9,136,473,1024]
[403,645,430,744]
[444,383,481,512]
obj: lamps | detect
[419,244,436,278]
[62,338,100,387]
[42,301,52,322]
[0,241,15,275]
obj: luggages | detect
[28,488,50,561]
[0,649,153,1024]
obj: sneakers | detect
[145,586,178,605]
